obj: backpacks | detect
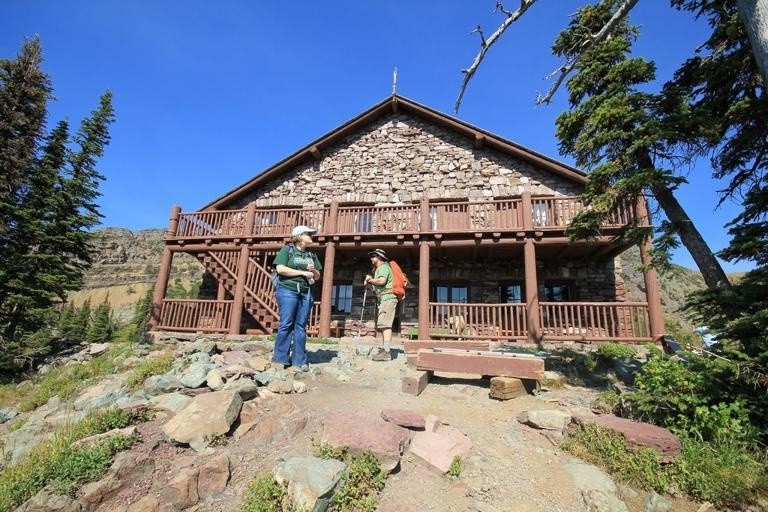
[268,243,312,289]
[383,260,409,302]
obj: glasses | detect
[304,232,312,236]
[371,256,379,258]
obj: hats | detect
[293,226,317,237]
[367,248,389,261]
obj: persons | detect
[363,248,398,361]
[271,224,323,366]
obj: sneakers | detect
[372,348,385,357]
[372,351,391,360]
